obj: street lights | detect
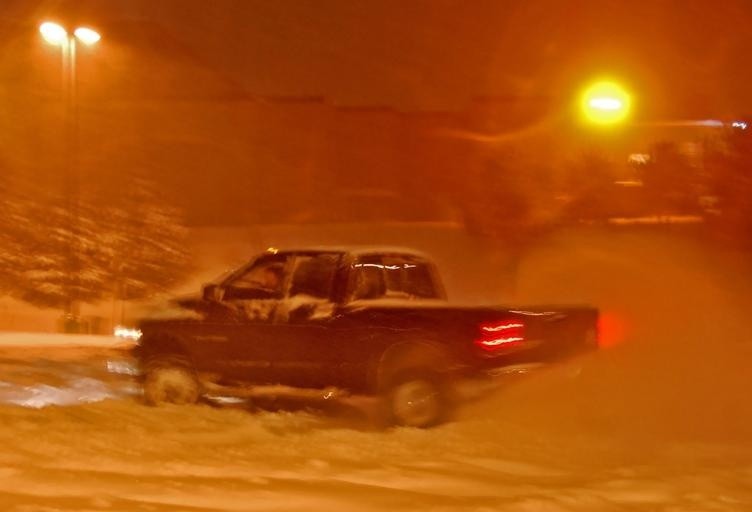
[38,15,104,332]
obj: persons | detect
[263,265,284,288]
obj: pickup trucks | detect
[115,244,619,427]
